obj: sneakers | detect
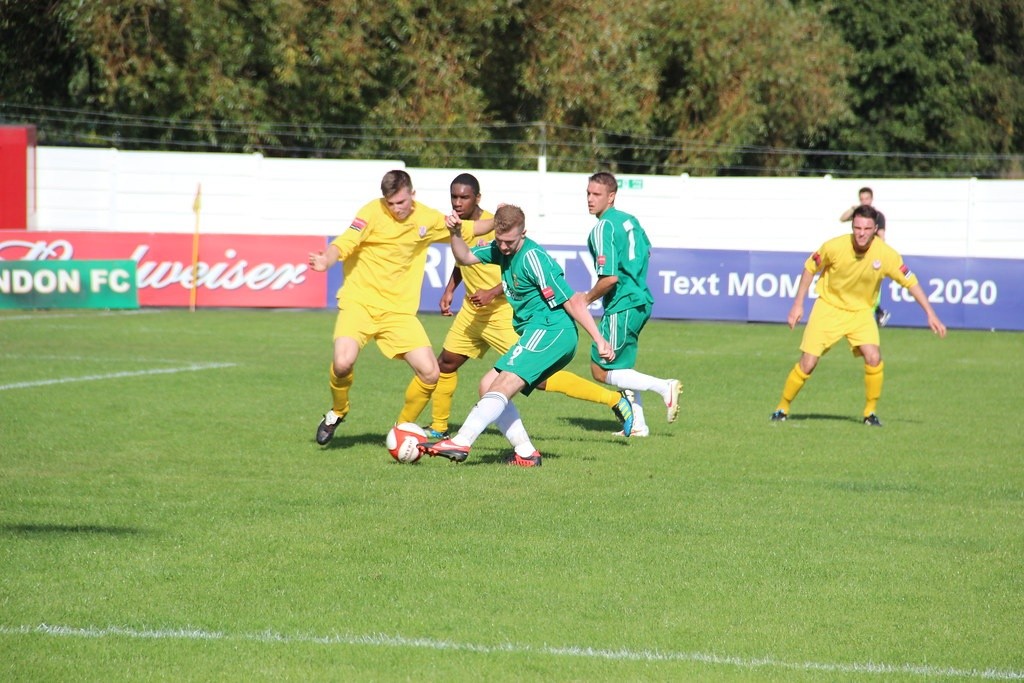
[769,410,787,425]
[314,406,348,449]
[878,309,890,327]
[501,450,542,468]
[417,423,449,441]
[611,388,635,438]
[661,378,684,424]
[863,412,883,427]
[609,426,648,437]
[414,436,470,465]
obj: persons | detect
[580,170,683,438]
[421,173,634,441]
[417,204,615,465]
[770,205,950,429]
[840,187,894,327]
[309,169,507,444]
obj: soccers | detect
[386,422,428,467]
